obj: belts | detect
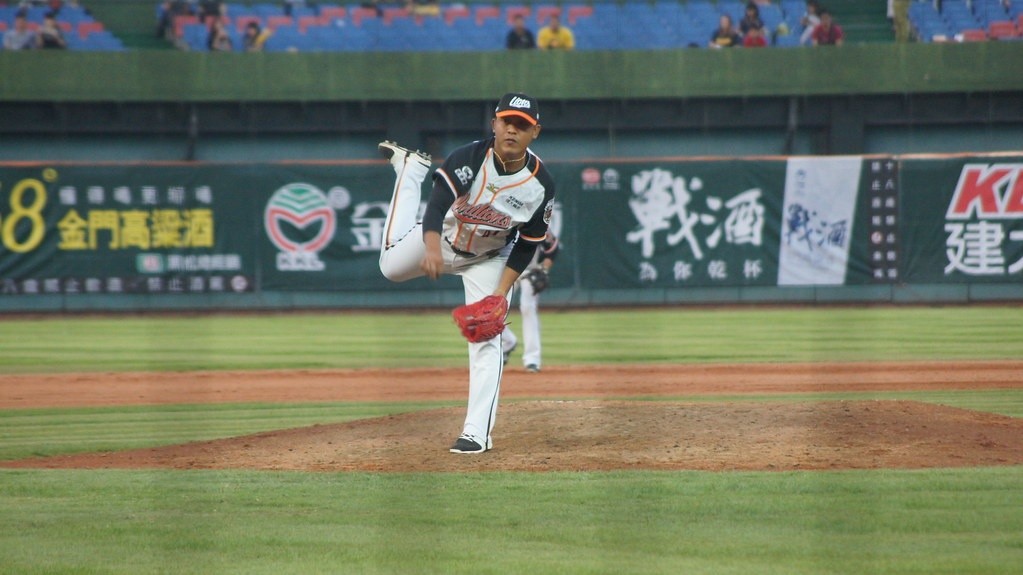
[444,236,475,258]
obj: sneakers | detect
[450,433,489,453]
[378,139,432,169]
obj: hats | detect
[495,92,539,126]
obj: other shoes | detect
[504,343,516,365]
[527,364,538,373]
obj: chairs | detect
[0,0,1023,51]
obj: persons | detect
[206,19,235,51]
[538,15,576,48]
[1,0,229,23]
[800,2,844,46]
[706,13,743,48]
[505,199,563,371]
[376,91,556,452]
[735,3,765,36]
[4,11,36,49]
[742,24,769,48]
[506,14,537,49]
[31,13,69,49]
[242,21,268,52]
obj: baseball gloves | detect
[521,267,550,295]
[452,295,509,343]
